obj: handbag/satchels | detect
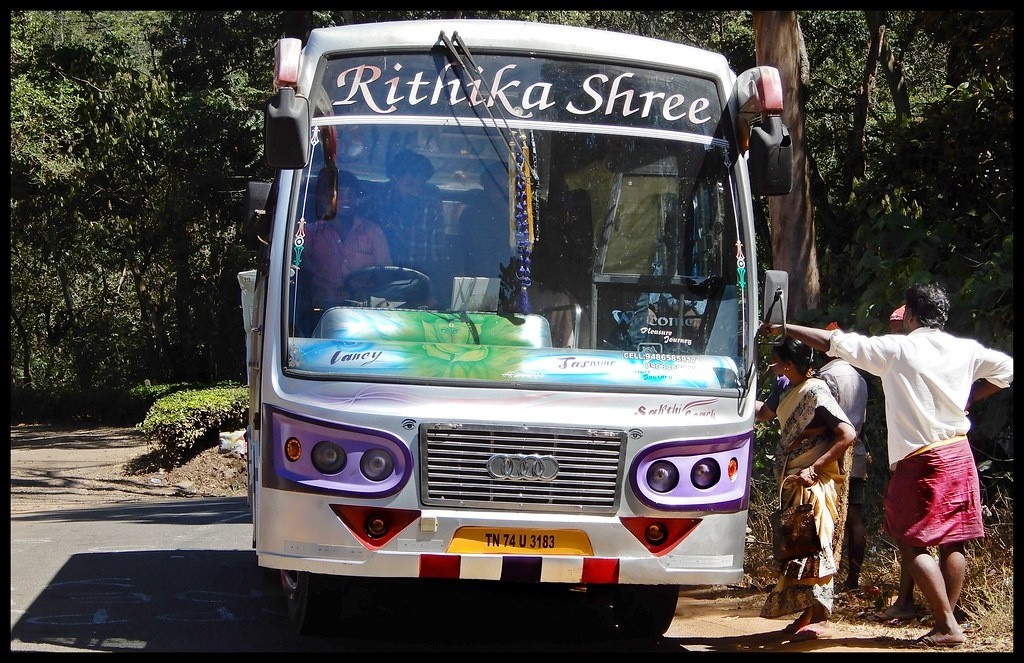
[768,474,823,563]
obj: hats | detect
[889,304,906,321]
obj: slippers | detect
[865,606,917,622]
[909,628,968,646]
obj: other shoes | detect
[842,580,861,592]
[787,630,818,642]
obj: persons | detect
[292,170,393,312]
[867,562,915,623]
[370,150,445,281]
[755,335,857,632]
[811,317,866,593]
[759,281,1014,647]
[458,162,512,278]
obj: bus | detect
[235,19,795,637]
